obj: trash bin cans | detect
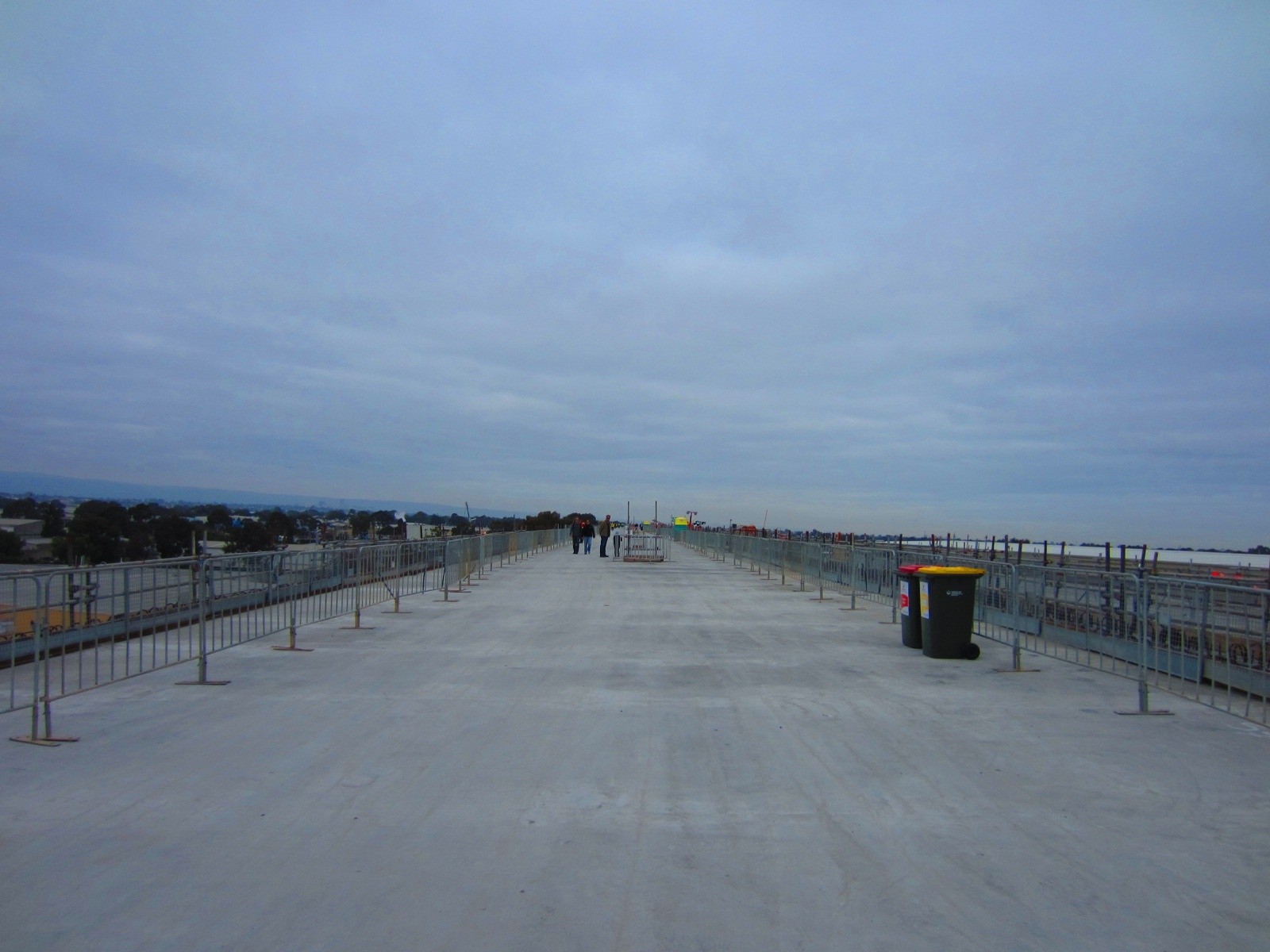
[674,518,687,541]
[892,565,945,649]
[913,566,985,659]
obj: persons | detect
[599,515,611,558]
[611,522,625,531]
[687,522,702,532]
[613,530,622,557]
[634,523,643,531]
[569,517,596,555]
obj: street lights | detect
[730,519,732,527]
[687,511,697,530]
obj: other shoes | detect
[600,554,609,557]
[573,551,578,554]
